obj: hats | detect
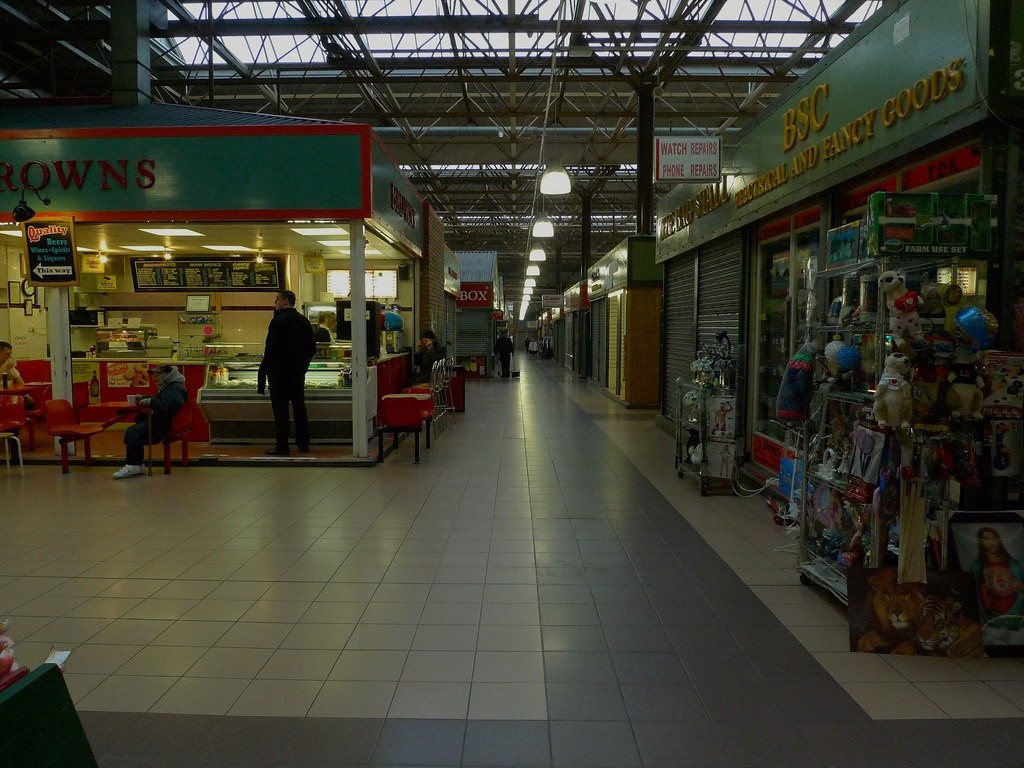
[147,363,174,374]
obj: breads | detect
[123,364,150,386]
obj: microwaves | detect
[69,310,104,327]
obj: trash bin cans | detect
[439,365,465,412]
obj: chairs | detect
[374,386,436,464]
[73,380,89,420]
[45,400,103,473]
[25,385,52,450]
[0,394,24,434]
[415,356,456,435]
[157,401,193,474]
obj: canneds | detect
[342,373,349,387]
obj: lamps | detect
[538,55,571,194]
[12,183,50,221]
[517,142,555,320]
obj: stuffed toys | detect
[946,346,987,419]
[878,271,928,353]
[873,353,913,429]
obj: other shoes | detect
[265,448,290,455]
[298,447,310,453]
[112,463,147,478]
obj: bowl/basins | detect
[127,395,142,405]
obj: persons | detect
[719,444,732,477]
[410,329,447,383]
[257,290,316,457]
[0,341,35,465]
[494,331,514,378]
[710,401,733,436]
[525,337,532,354]
[113,364,188,478]
[315,311,336,344]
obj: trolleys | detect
[667,379,742,496]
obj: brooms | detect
[496,354,501,375]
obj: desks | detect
[0,380,51,395]
[87,400,148,418]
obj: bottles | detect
[91,371,99,397]
[3,370,7,388]
[211,367,229,385]
[338,368,352,387]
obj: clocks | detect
[21,278,36,296]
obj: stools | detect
[0,432,24,477]
